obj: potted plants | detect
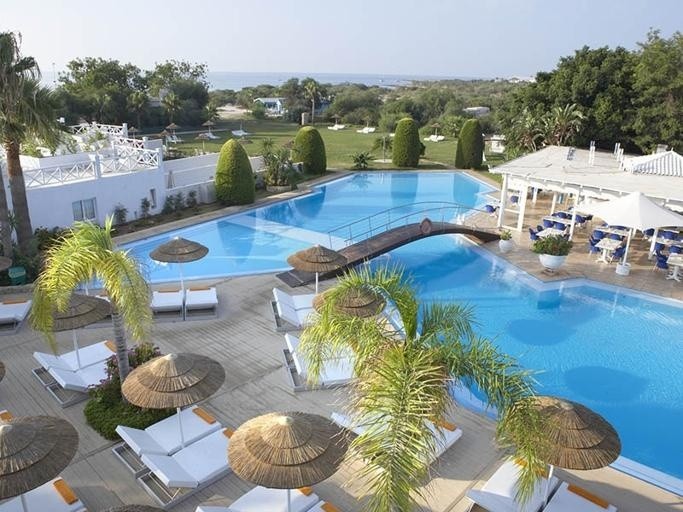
[260,137,295,195]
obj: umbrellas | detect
[201,120,216,133]
[157,130,174,151]
[577,190,682,265]
[280,138,295,149]
[1,413,78,510]
[312,284,387,323]
[29,291,111,368]
[228,410,347,512]
[290,244,348,293]
[329,114,340,124]
[361,114,373,127]
[429,123,442,135]
[195,133,211,153]
[166,123,181,134]
[121,351,225,447]
[128,127,142,140]
[149,236,209,289]
[506,395,621,509]
[238,136,253,146]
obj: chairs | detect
[150,286,218,322]
[32,341,116,409]
[269,287,322,331]
[467,454,617,512]
[484,187,682,285]
[195,486,338,512]
[327,122,446,143]
[280,332,363,394]
[161,127,253,145]
[1,298,32,337]
[1,407,88,512]
[110,403,236,510]
[331,410,464,483]
